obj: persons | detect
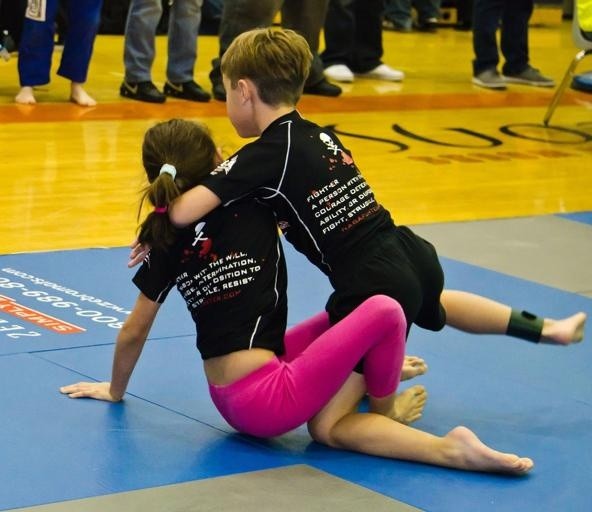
[1,0,557,106]
[59,117,428,439]
[128,27,586,475]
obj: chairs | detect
[543,0,592,127]
